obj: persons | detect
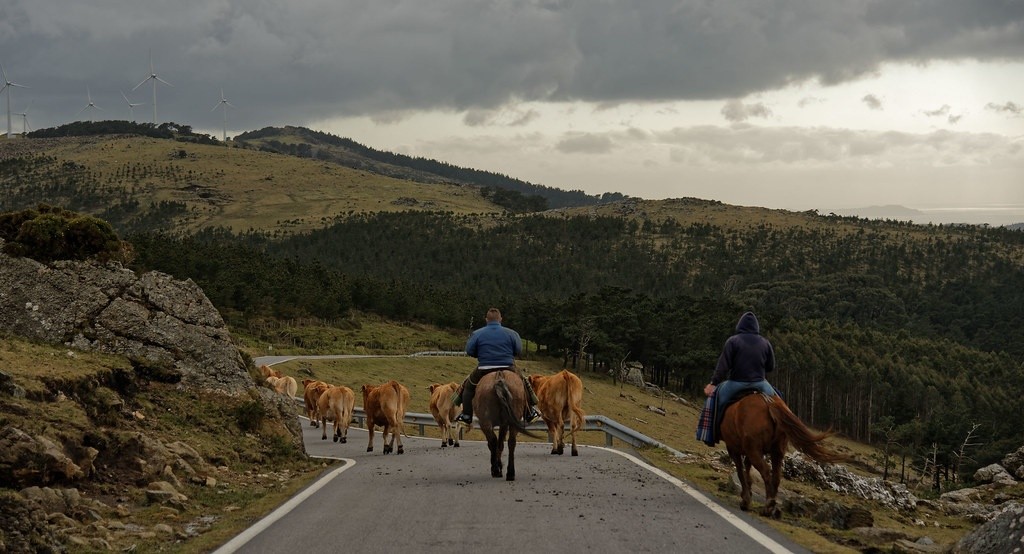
[697,311,776,446]
[456,307,540,426]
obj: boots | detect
[523,378,539,425]
[458,376,477,424]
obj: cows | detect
[257,363,587,456]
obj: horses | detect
[717,392,843,517]
[469,369,551,483]
